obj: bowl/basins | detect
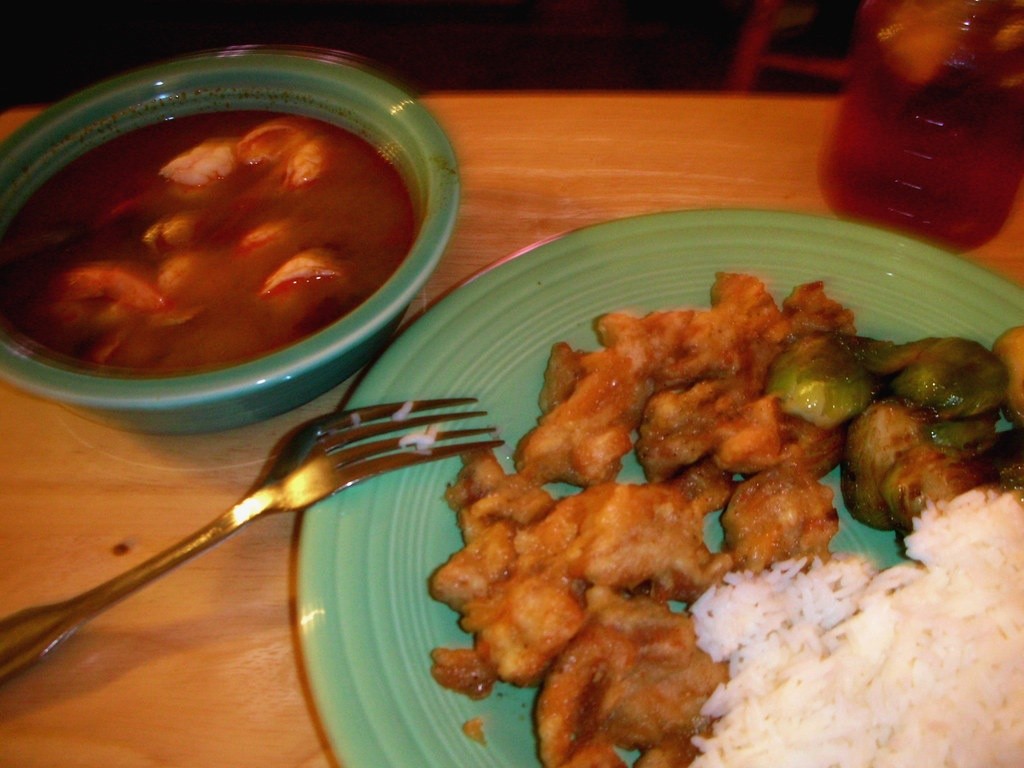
[0,49,460,435]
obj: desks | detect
[0,86,1023,768]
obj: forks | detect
[0,396,507,682]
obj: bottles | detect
[815,0,1024,256]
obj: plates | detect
[293,207,1024,768]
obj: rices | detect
[684,488,1024,768]
[350,401,442,451]
